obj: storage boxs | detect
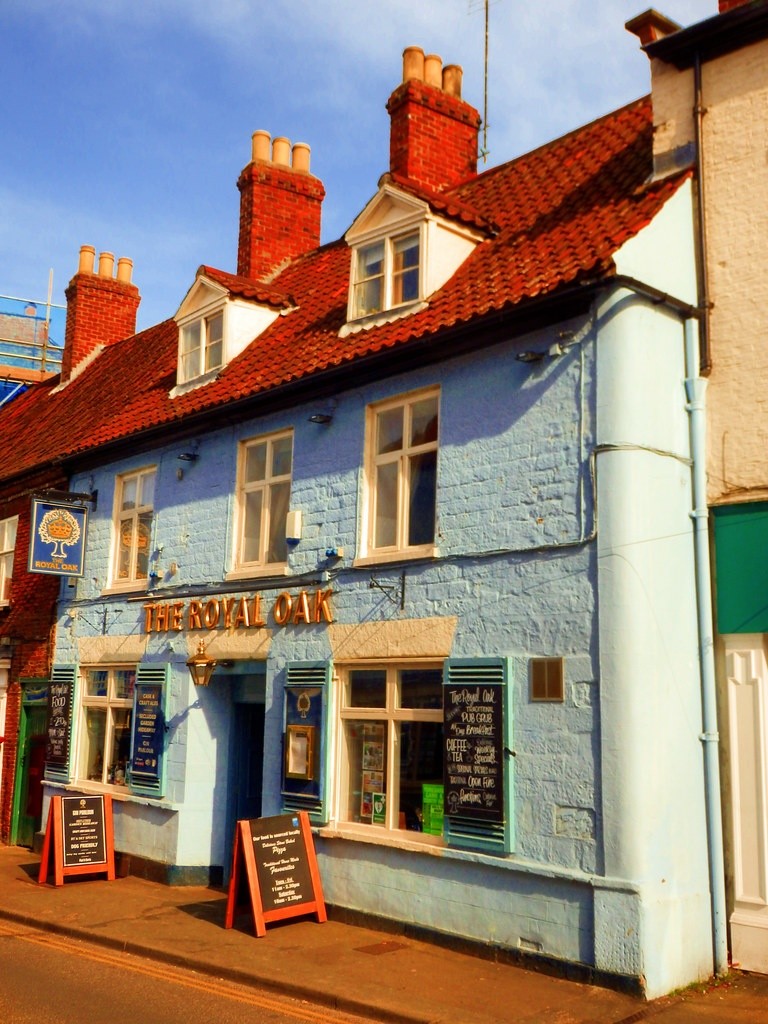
[422,783,444,833]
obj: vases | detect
[114,769,124,785]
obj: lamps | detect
[177,453,200,461]
[515,350,547,362]
[186,639,234,685]
[306,413,333,423]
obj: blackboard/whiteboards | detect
[43,681,72,766]
[39,794,116,874]
[442,683,505,825]
[228,810,324,923]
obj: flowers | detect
[105,762,128,773]
